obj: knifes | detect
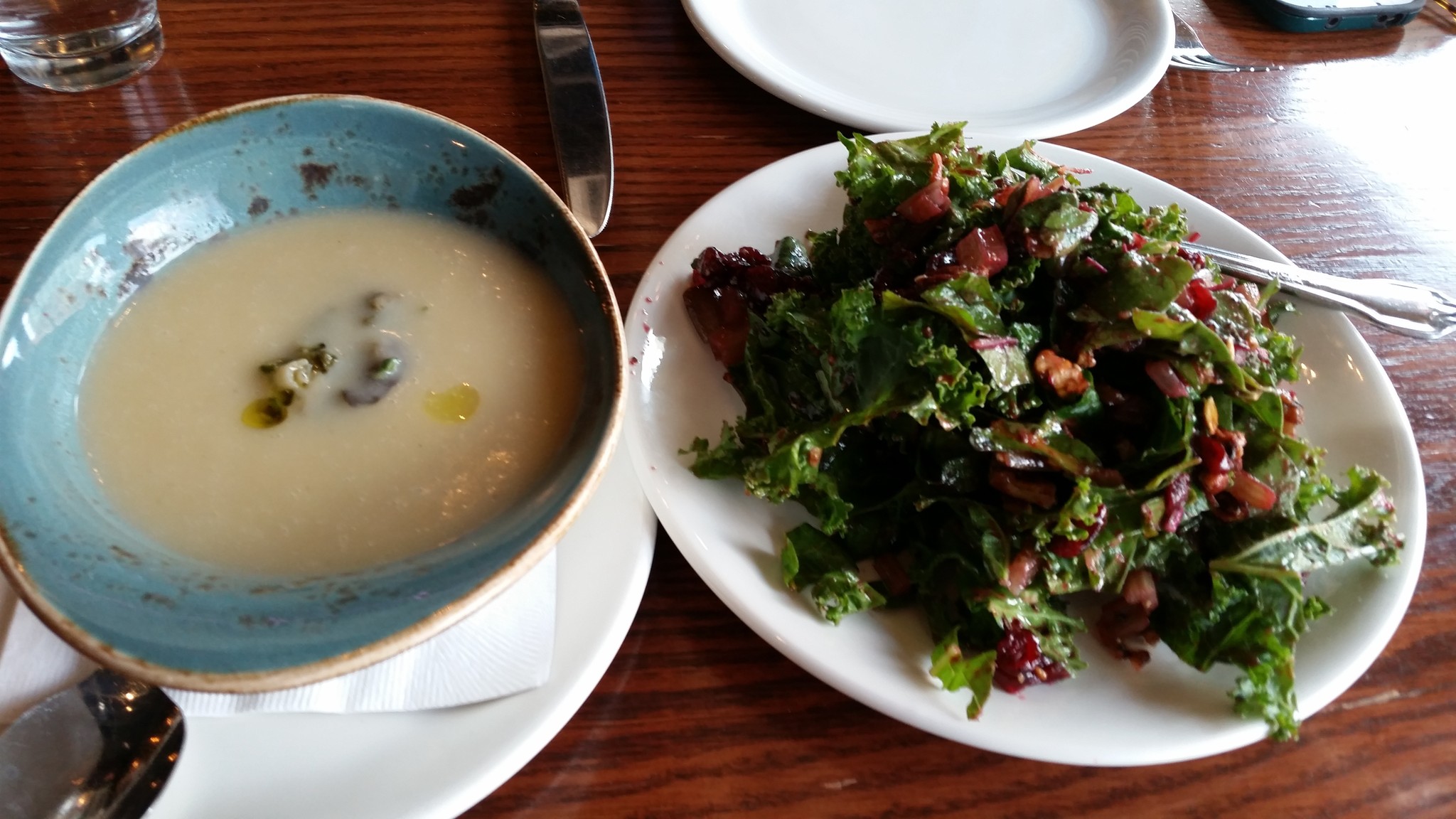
[529,0,613,239]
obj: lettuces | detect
[675,128,1407,744]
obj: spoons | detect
[0,670,185,819]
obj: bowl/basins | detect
[0,92,621,694]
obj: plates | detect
[620,132,1429,766]
[671,0,1176,139]
[0,429,658,819]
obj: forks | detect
[1171,7,1286,73]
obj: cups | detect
[0,0,165,91]
